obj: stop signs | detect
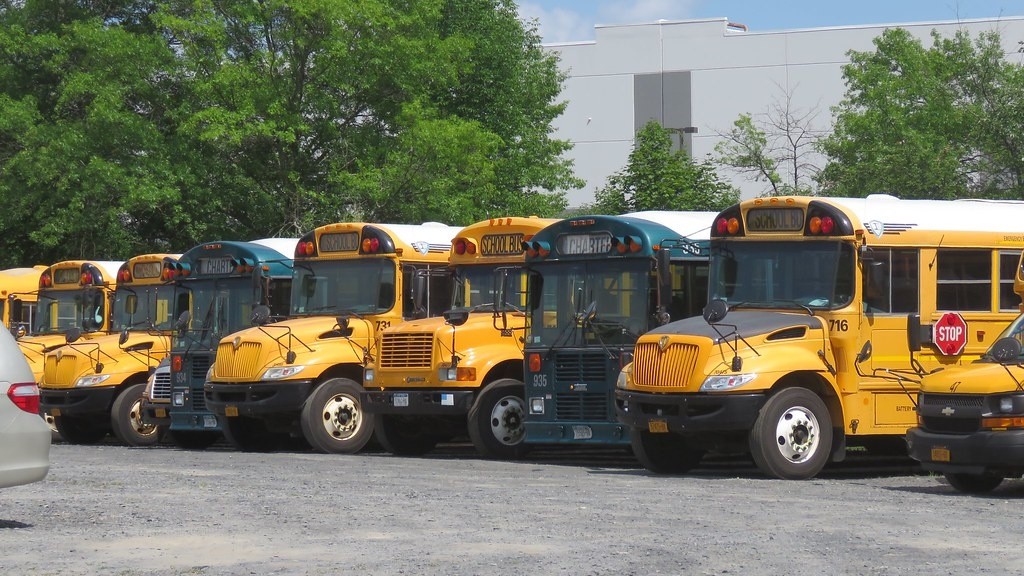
[932,311,969,357]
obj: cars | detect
[0,320,51,486]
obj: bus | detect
[492,210,723,449]
[906,247,1024,496]
[615,193,1023,481]
[0,215,711,461]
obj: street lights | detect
[664,126,698,155]
